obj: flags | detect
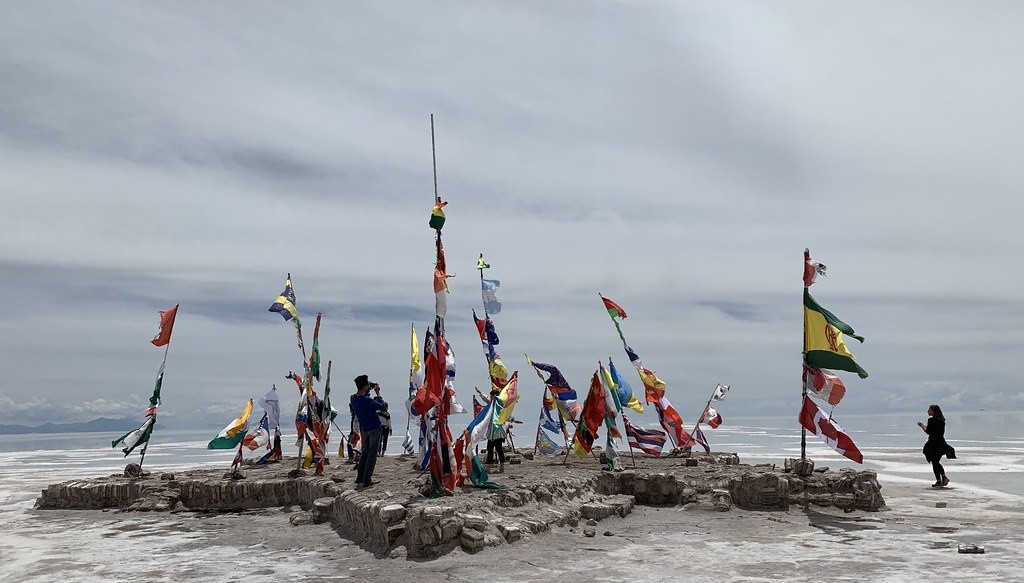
[477,257,490,270]
[462,309,518,488]
[403,322,468,497]
[533,359,579,455]
[112,360,168,457]
[799,257,869,464]
[480,279,502,315]
[601,296,628,319]
[268,280,299,321]
[690,382,730,455]
[294,360,338,476]
[150,308,179,347]
[430,197,456,284]
[310,311,329,381]
[210,384,282,471]
[338,430,362,463]
[572,346,695,470]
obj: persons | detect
[485,389,507,474]
[346,375,392,488]
[434,263,456,320]
[918,404,956,487]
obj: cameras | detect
[370,383,377,389]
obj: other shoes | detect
[364,480,380,485]
[942,477,950,486]
[932,481,942,486]
